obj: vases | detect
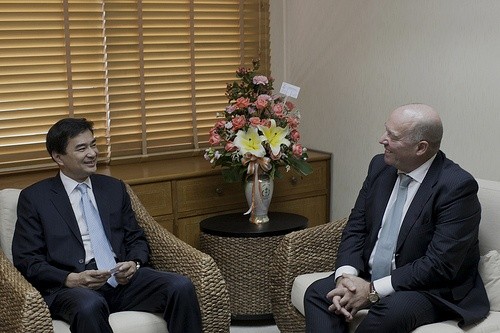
[245,175,274,224]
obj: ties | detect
[77,184,118,288]
[372,174,413,282]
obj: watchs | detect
[135,260,142,269]
[367,282,378,303]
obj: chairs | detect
[0,183,229,333]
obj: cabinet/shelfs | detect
[130,159,331,253]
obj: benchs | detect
[268,178,500,333]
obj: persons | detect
[303,103,491,333]
[12,118,203,333]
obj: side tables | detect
[200,211,309,325]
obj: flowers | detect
[204,59,313,225]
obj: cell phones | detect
[102,264,122,274]
